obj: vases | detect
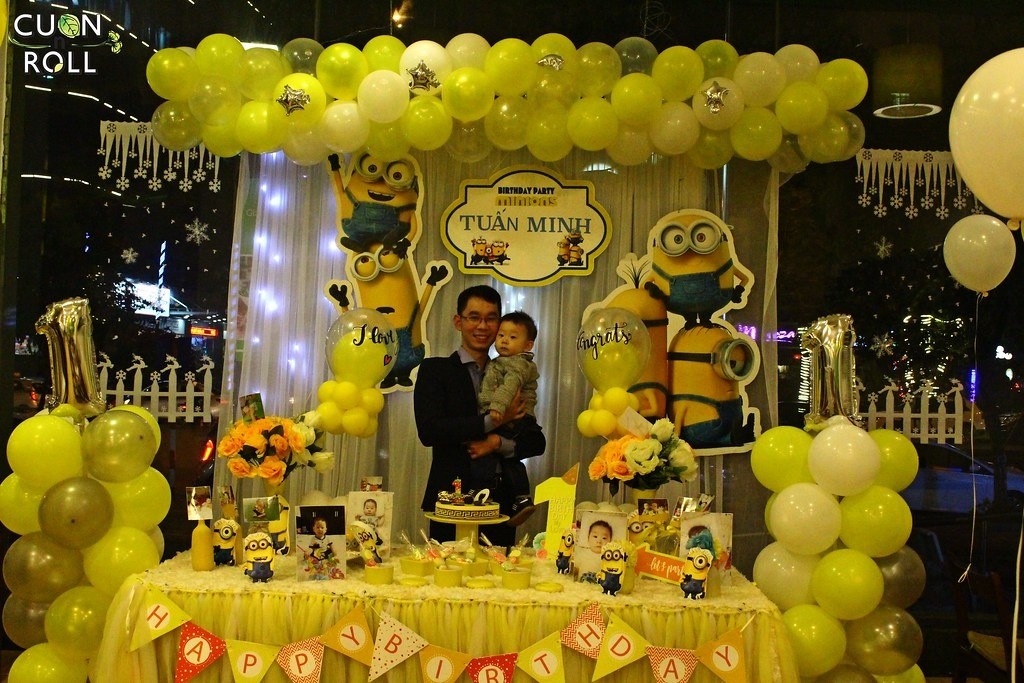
[632,490,658,507]
[259,478,288,548]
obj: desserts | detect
[354,529,530,572]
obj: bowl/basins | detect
[365,541,531,588]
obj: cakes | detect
[435,478,499,520]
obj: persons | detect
[355,499,386,546]
[413,285,547,556]
[642,502,664,516]
[201,497,212,509]
[252,499,266,518]
[308,519,339,564]
[577,519,614,580]
[477,308,540,526]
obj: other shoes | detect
[506,496,536,526]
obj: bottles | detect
[191,520,213,571]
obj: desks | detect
[95,534,799,683]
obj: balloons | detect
[944,48,1024,296]
[0,404,172,683]
[146,30,870,170]
[317,307,399,438]
[751,425,927,683]
[577,305,651,438]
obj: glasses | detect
[460,314,503,328]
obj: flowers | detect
[219,406,329,520]
[588,413,700,487]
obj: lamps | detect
[872,9,944,119]
